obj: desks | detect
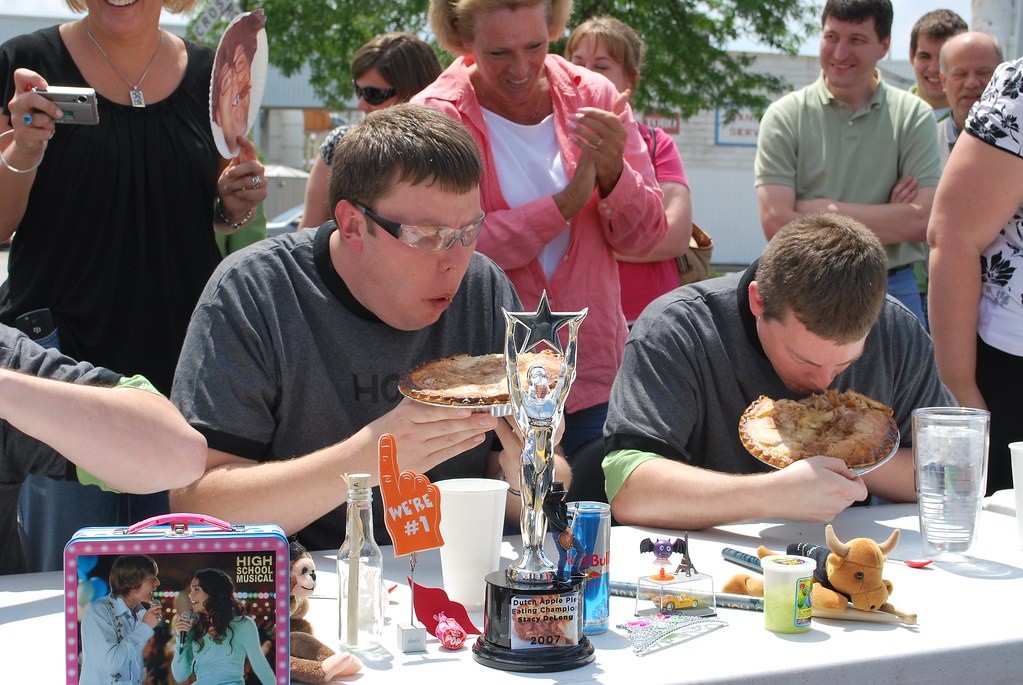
[1,486,1023,685]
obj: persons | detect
[1,320,210,574]
[904,8,1003,340]
[755,0,942,339]
[168,104,572,556]
[78,554,277,685]
[0,1,267,533]
[601,213,964,532]
[927,59,1023,499]
[299,0,692,519]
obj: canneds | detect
[565,501,611,635]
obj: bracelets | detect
[216,197,252,230]
[0,127,48,174]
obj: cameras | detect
[30,86,99,124]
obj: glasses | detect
[353,79,396,107]
[343,196,486,252]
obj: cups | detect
[1008,442,1023,551]
[912,406,990,561]
[432,478,509,607]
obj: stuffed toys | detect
[290,541,362,685]
[722,525,900,614]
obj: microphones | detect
[179,612,191,655]
[152,600,160,607]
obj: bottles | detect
[336,474,384,654]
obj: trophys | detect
[473,289,596,673]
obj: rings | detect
[22,111,34,128]
[252,174,261,190]
[594,138,603,147]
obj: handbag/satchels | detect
[674,224,714,285]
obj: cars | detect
[262,164,312,236]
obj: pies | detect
[739,388,898,471]
[399,349,569,408]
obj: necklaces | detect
[85,24,163,108]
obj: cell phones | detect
[16,307,54,340]
